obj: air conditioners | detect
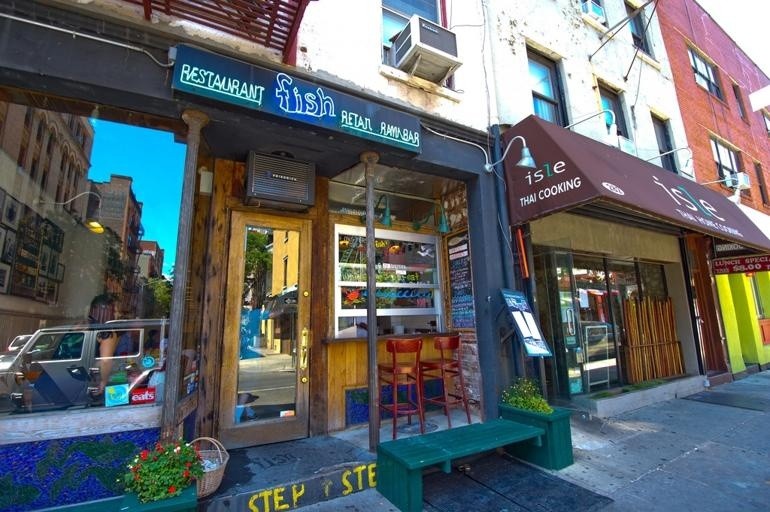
[726,172,750,190]
[245,155,316,210]
[389,14,464,83]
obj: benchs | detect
[376,417,546,512]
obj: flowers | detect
[116,436,204,503]
[505,379,555,414]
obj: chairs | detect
[376,338,425,440]
[421,336,472,429]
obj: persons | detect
[112,325,141,354]
[26,291,122,409]
[144,329,168,349]
[236,293,296,422]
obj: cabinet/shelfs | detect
[334,228,445,338]
[582,323,610,392]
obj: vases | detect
[501,404,574,469]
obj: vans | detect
[1,318,170,414]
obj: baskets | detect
[187,436,230,498]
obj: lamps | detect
[483,134,537,179]
[33,192,103,240]
[362,196,393,226]
[639,143,697,177]
[565,108,620,150]
[413,200,451,234]
[701,178,742,203]
